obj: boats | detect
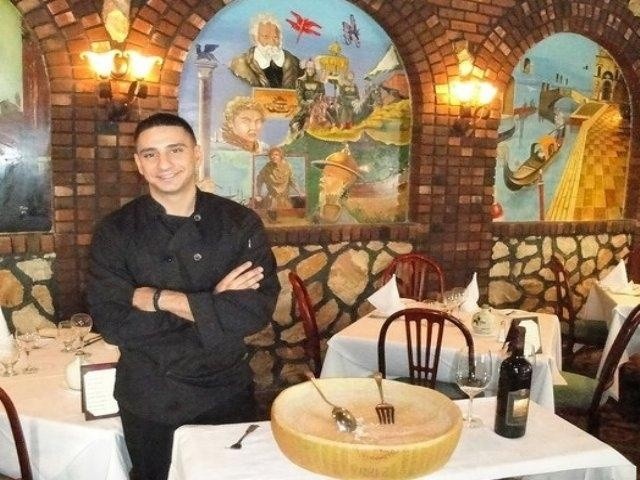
[499,123,567,195]
[513,103,537,120]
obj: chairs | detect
[550,303,640,439]
[383,254,444,303]
[376,307,485,400]
[288,272,322,378]
[543,255,608,363]
[0,388,33,480]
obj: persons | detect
[255,144,303,211]
[308,143,366,224]
[336,71,361,129]
[289,58,327,129]
[230,13,304,89]
[87,117,281,480]
[221,96,269,153]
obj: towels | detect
[461,271,479,311]
[366,273,405,314]
[600,258,632,295]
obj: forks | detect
[373,370,394,423]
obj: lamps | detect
[77,47,164,124]
[450,79,498,138]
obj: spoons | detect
[299,363,357,433]
[225,424,260,449]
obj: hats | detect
[310,147,367,183]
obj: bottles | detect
[470,303,496,335]
[494,323,534,440]
[63,352,95,391]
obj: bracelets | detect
[152,288,162,312]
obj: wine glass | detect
[441,286,467,326]
[58,313,94,358]
[0,326,40,378]
[455,344,492,432]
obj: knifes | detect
[76,334,106,349]
